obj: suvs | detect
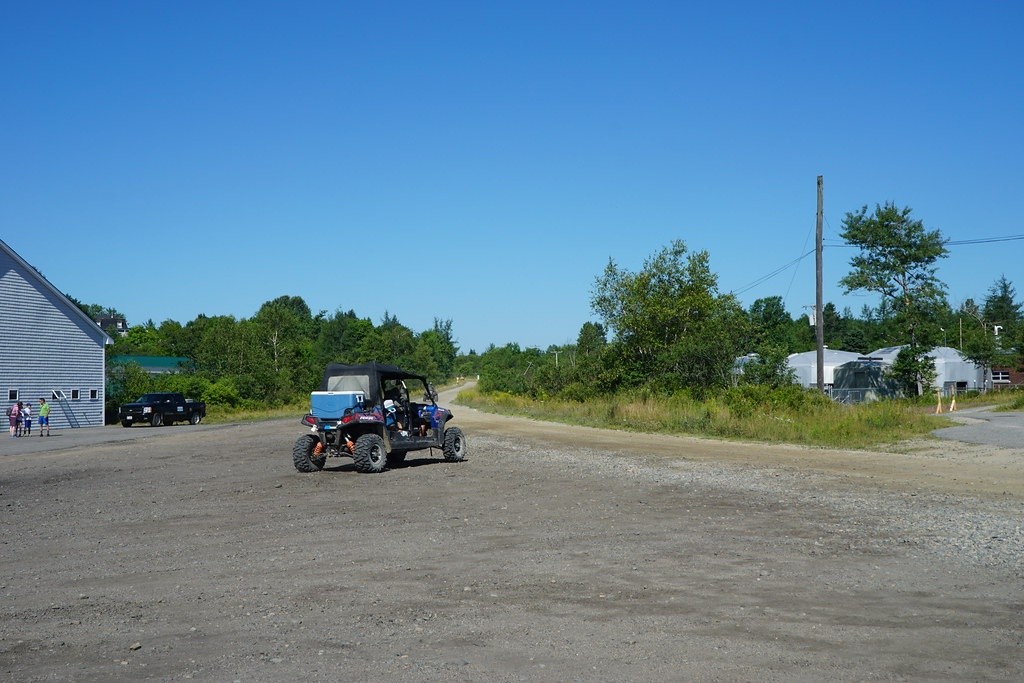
[292,362,467,472]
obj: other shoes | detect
[28,434,32,437]
[40,434,43,437]
[420,433,427,437]
[23,434,28,437]
[47,434,49,436]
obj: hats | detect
[27,403,33,406]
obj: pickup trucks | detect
[118,392,206,428]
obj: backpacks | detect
[6,406,18,416]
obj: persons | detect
[38,398,50,437]
[428,381,434,390]
[9,402,32,437]
